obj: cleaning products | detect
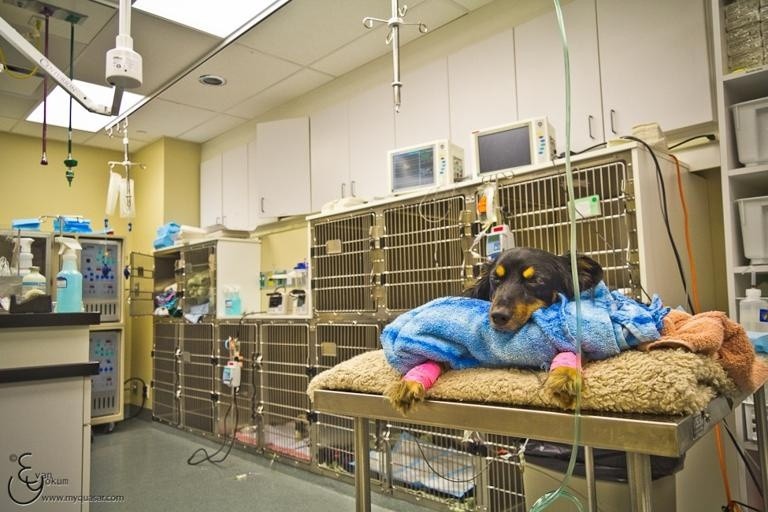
[738,288,768,330]
[10,237,34,274]
[54,236,83,312]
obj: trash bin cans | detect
[521,440,684,512]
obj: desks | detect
[0,303,101,511]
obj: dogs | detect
[389,246,651,419]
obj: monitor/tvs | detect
[388,139,464,196]
[472,115,556,179]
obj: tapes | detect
[25,289,47,300]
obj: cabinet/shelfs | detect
[708,0,768,509]
[393,29,516,182]
[247,118,312,225]
[309,85,395,211]
[121,148,734,508]
[514,0,717,155]
